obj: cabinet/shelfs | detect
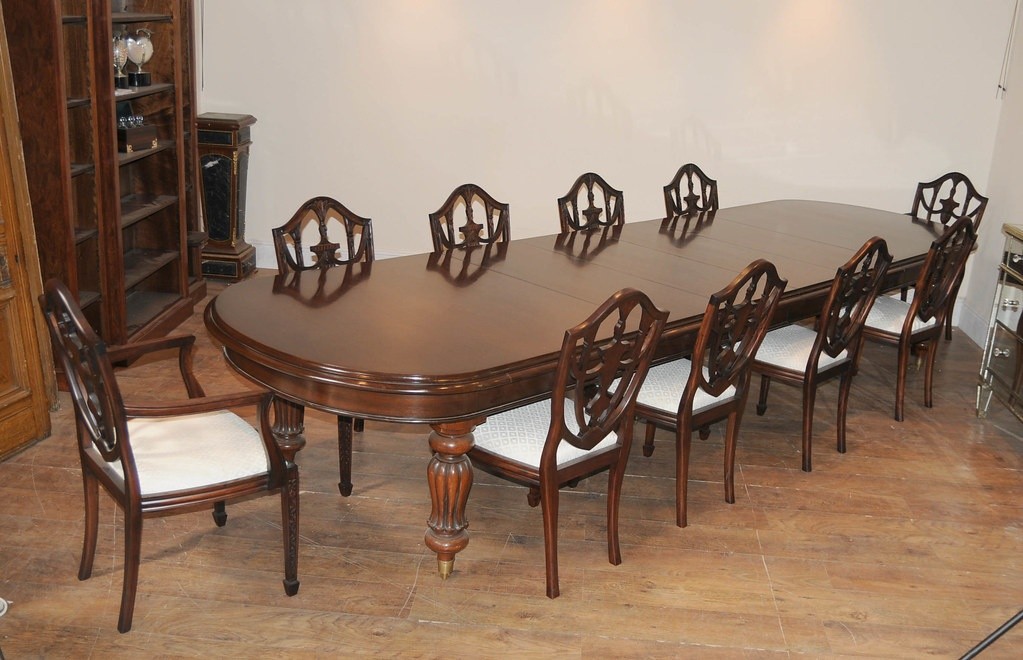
[0,0,208,392]
[976,223,1023,424]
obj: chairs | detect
[815,216,979,422]
[659,210,716,249]
[557,172,625,233]
[900,172,989,340]
[428,184,511,250]
[426,241,509,288]
[271,196,377,433]
[38,285,300,633]
[467,287,671,599]
[722,235,894,473]
[595,259,789,528]
[553,225,622,266]
[271,261,372,309]
[663,163,719,217]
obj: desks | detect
[202,200,978,580]
